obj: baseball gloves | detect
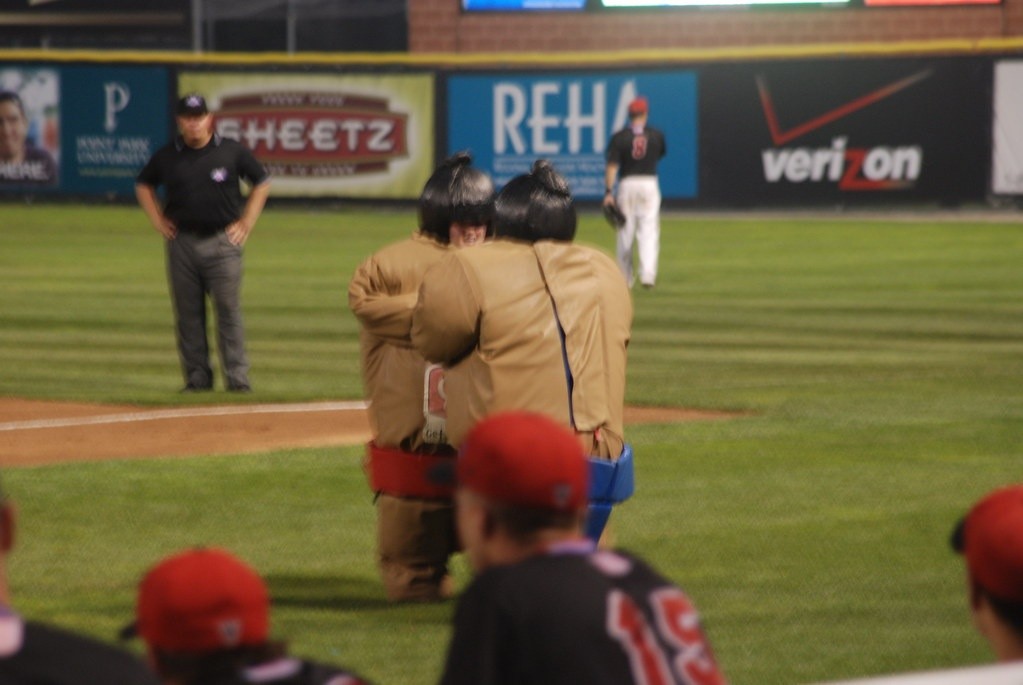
[603,204,625,228]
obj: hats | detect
[116,549,271,653]
[627,99,647,116]
[951,485,1023,598]
[455,410,589,512]
[175,95,206,117]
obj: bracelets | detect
[606,188,612,193]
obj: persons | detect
[134,93,273,394]
[118,543,376,685]
[408,159,634,577]
[949,484,1022,664]
[347,153,495,607]
[603,99,667,291]
[435,409,726,685]
[0,92,57,181]
[0,488,153,685]
